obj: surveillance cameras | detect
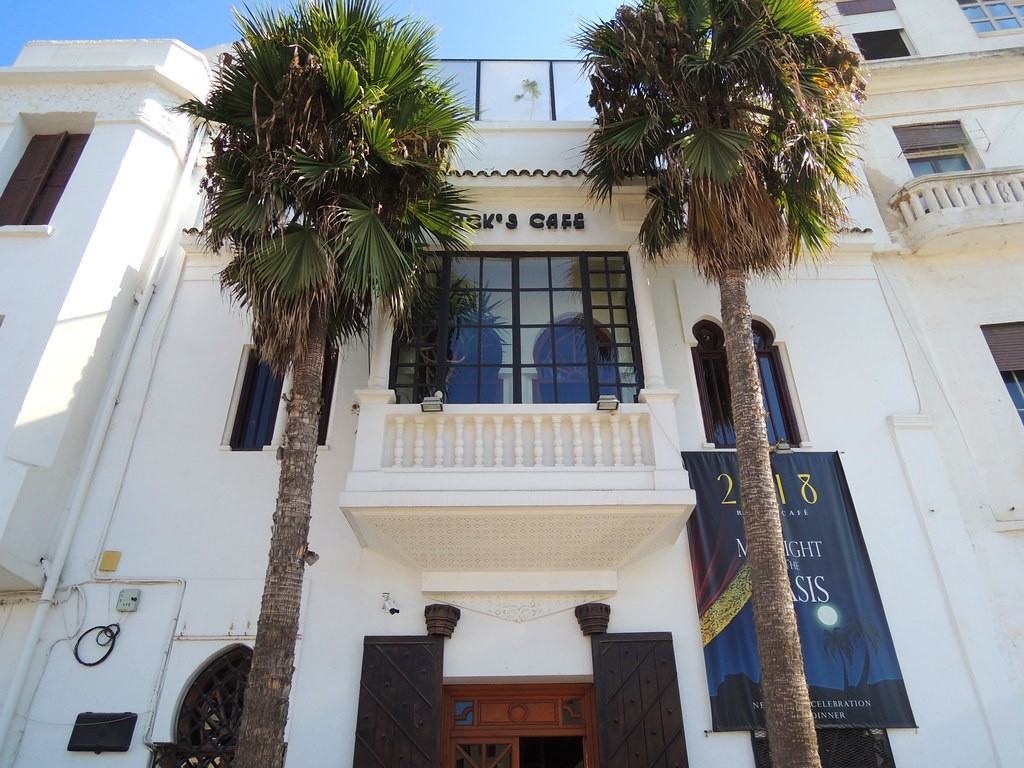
[385,600,400,615]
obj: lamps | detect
[595,393,620,412]
[419,391,445,414]
